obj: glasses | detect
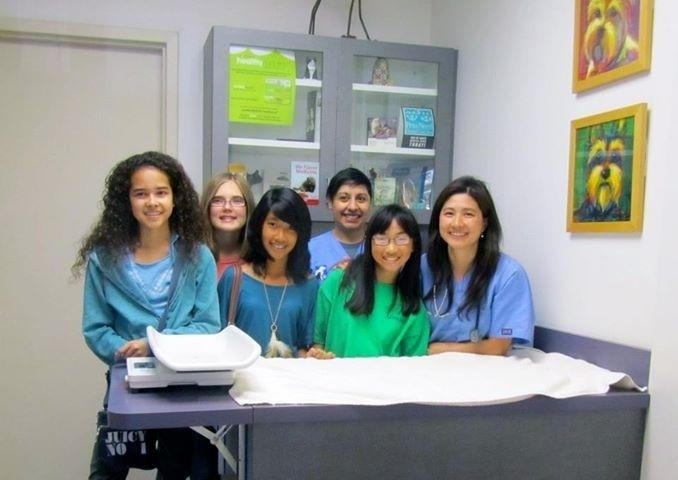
[371,234,413,248]
[210,196,248,209]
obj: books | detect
[260,87,437,212]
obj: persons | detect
[194,170,256,284]
[309,202,433,360]
[307,166,375,285]
[216,184,322,359]
[418,173,535,356]
[81,149,225,480]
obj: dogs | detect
[572,118,630,223]
[582,0,640,80]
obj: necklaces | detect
[258,265,289,333]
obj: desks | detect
[93,348,651,479]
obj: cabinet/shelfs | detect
[202,23,463,227]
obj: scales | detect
[125,328,262,394]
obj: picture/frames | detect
[569,0,657,95]
[566,103,652,236]
